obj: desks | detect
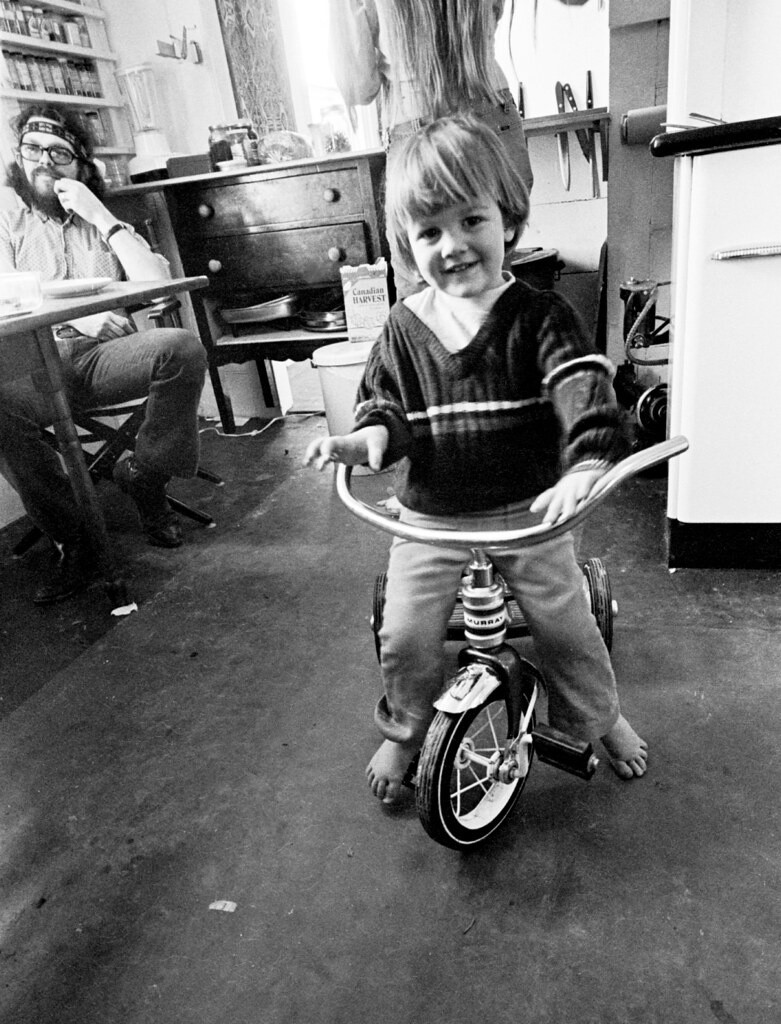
[0,276,210,609]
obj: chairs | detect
[13,218,222,557]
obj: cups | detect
[320,104,352,153]
[209,122,260,170]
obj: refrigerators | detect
[650,0,781,568]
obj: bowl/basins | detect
[0,271,43,318]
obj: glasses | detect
[19,143,79,166]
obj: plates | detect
[41,278,113,298]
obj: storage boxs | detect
[167,154,213,179]
[339,257,390,343]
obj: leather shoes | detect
[111,456,184,549]
[34,557,118,605]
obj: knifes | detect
[518,81,528,149]
[564,84,591,163]
[555,82,570,191]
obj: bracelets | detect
[102,223,127,244]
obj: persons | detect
[0,103,207,605]
[299,115,648,807]
[339,1,534,308]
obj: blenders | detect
[112,62,172,184]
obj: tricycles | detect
[333,433,690,853]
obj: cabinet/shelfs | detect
[103,150,399,434]
[0,0,136,163]
